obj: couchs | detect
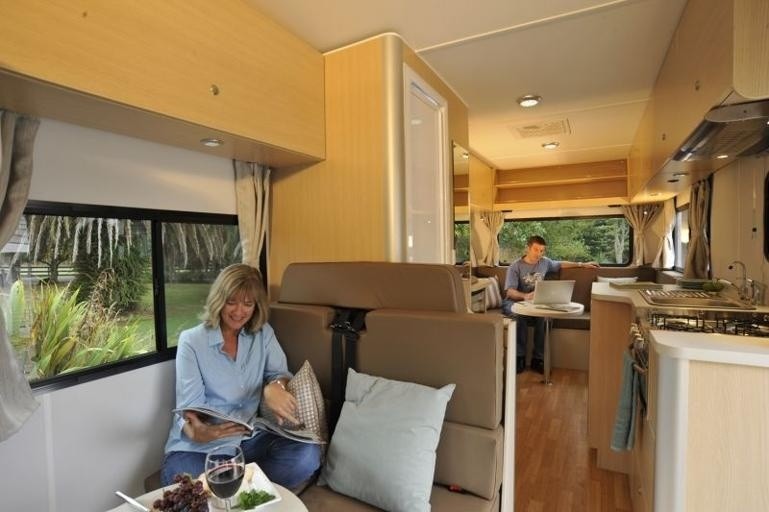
[471,268,676,372]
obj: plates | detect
[207,461,282,510]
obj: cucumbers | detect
[703,283,724,292]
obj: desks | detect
[511,302,584,384]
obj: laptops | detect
[525,280,577,305]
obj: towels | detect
[611,350,639,452]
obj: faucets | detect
[727,259,749,299]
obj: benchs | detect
[145,262,504,512]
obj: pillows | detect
[260,359,330,465]
[479,275,502,309]
[316,368,456,512]
[597,276,639,282]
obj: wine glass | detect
[205,445,246,512]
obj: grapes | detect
[153,475,209,512]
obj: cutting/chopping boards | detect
[610,281,662,289]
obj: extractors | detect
[673,99,768,161]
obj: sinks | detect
[650,297,755,310]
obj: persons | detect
[153,263,324,494]
[502,235,600,374]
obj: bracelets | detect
[269,378,287,391]
[577,261,582,268]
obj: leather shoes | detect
[531,358,544,374]
[516,356,526,373]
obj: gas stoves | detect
[627,312,769,366]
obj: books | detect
[171,406,330,446]
